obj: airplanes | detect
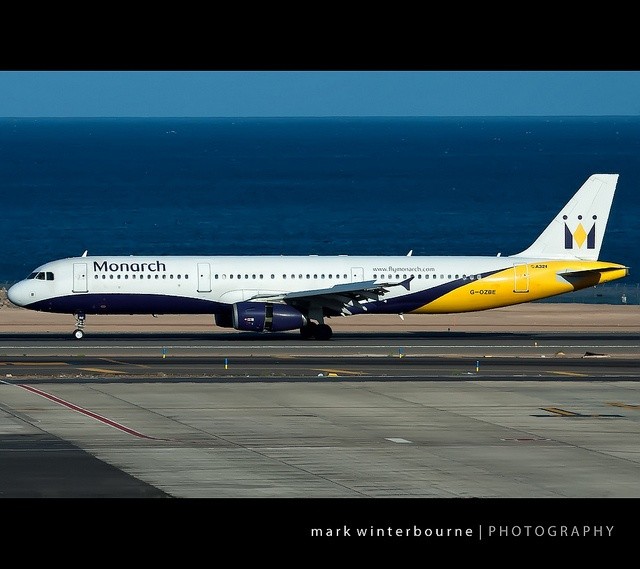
[7,172,631,339]
